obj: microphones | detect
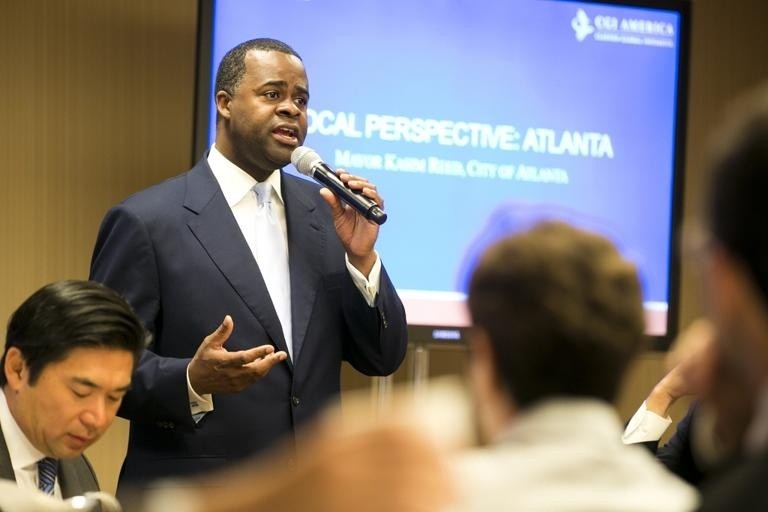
[289,144,387,223]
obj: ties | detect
[37,457,59,495]
[250,182,274,215]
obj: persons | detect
[619,322,742,497]
[443,220,705,511]
[697,113,768,511]
[88,38,408,511]
[0,279,144,512]
